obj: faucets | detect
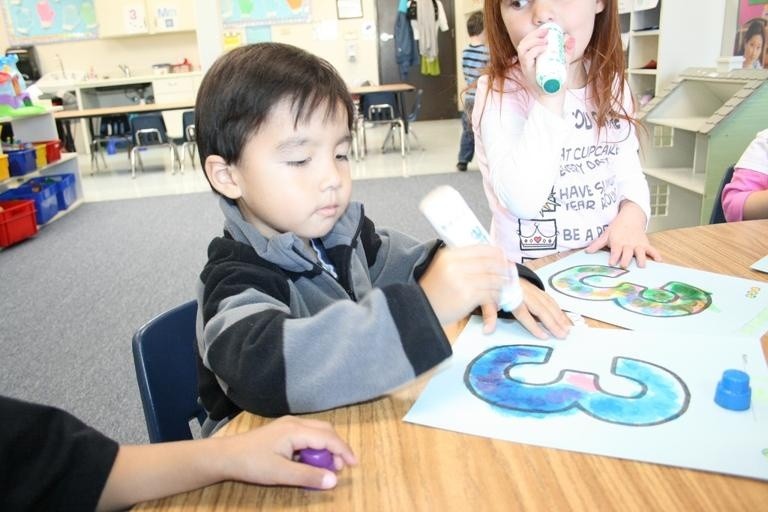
[118,64,130,78]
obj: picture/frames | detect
[335,1,364,20]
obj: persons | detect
[718,125,767,223]
[456,12,491,171]
[729,24,764,70]
[192,41,572,445]
[0,391,358,512]
[470,0,661,272]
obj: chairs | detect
[174,113,200,173]
[385,88,424,150]
[128,114,183,176]
[90,114,144,177]
[357,93,405,157]
[129,296,210,451]
[126,220,768,511]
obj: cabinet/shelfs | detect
[634,69,768,227]
[616,1,722,117]
[46,74,201,154]
[0,98,83,239]
[97,1,196,43]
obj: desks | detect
[54,81,416,165]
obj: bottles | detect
[419,182,526,312]
[533,23,572,93]
[708,370,756,458]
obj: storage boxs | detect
[26,173,78,210]
[1,184,60,224]
[1,199,38,249]
[0,138,63,180]
